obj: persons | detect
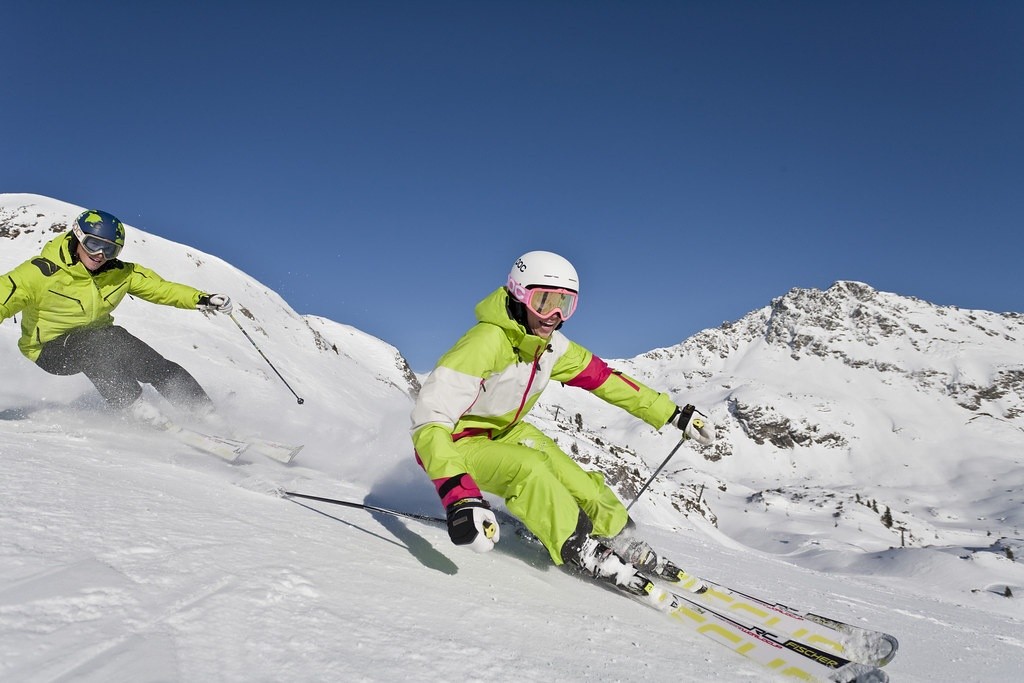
[410,250,717,610]
[0,210,232,435]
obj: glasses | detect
[508,274,579,321]
[73,220,123,259]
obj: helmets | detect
[76,210,125,246]
[508,251,579,303]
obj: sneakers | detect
[607,537,657,572]
[122,397,173,431]
[569,534,627,581]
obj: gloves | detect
[446,498,499,554]
[671,405,715,443]
[196,294,233,320]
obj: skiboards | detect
[157,406,302,469]
[485,497,898,682]
